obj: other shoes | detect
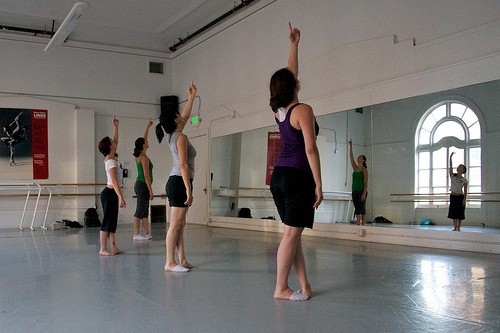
[133,234,146,240]
[146,234,152,240]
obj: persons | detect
[349,138,368,225]
[156,81,198,271]
[133,117,153,239]
[269,24,324,301]
[447,152,468,231]
[98,114,127,256]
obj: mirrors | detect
[208,80,500,235]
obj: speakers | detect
[160,95,179,133]
